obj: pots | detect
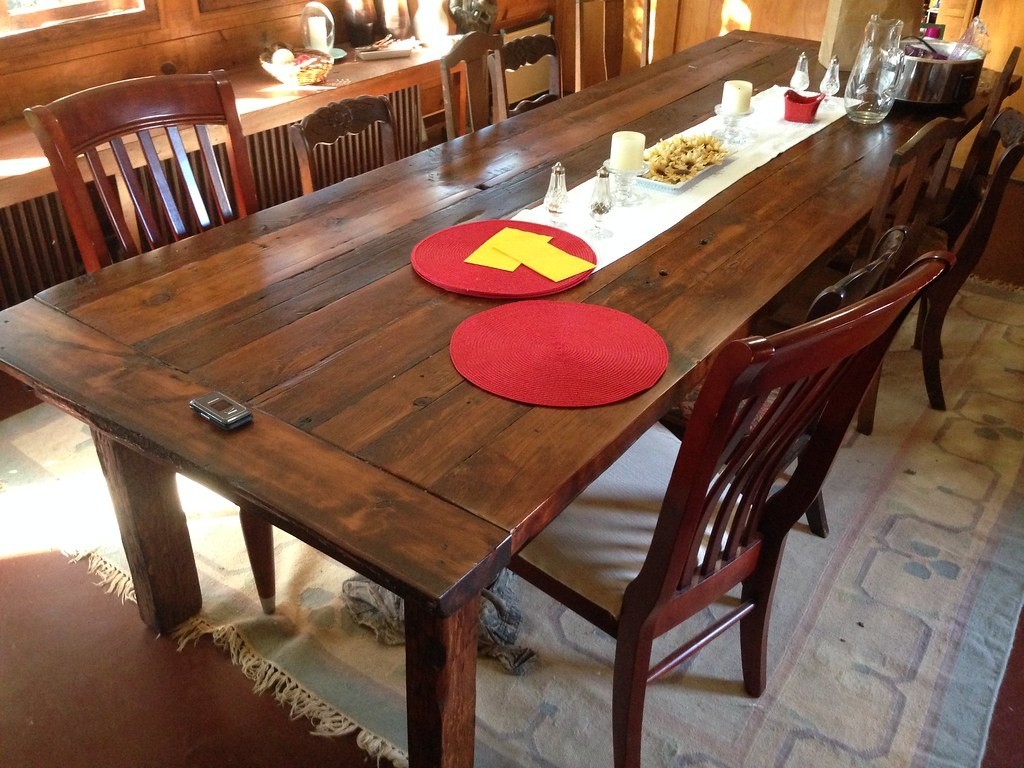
[893,37,985,117]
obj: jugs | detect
[843,14,906,126]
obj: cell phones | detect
[188,391,253,430]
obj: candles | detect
[611,131,646,170]
[721,80,752,113]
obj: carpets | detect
[34,271,1024,767]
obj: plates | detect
[354,46,412,59]
[637,147,738,193]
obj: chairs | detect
[291,95,395,194]
[507,43,1024,767]
[440,31,564,143]
[23,68,280,616]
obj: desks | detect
[1,29,1022,768]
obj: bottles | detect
[819,56,841,108]
[586,165,613,237]
[543,162,569,228]
[790,51,810,98]
[343,0,450,48]
